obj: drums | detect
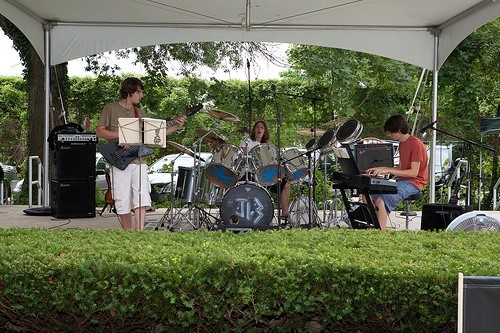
[336,120,364,144]
[305,138,317,152]
[175,166,225,204]
[248,144,286,187]
[318,130,337,153]
[219,181,275,228]
[281,149,309,183]
[205,143,244,188]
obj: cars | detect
[349,136,452,183]
[147,152,214,203]
[0,162,39,196]
[95,168,109,189]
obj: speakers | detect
[51,143,96,218]
[421,204,473,232]
[353,143,393,174]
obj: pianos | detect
[331,172,398,231]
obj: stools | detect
[401,191,424,231]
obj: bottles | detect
[83,117,92,133]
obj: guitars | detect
[99,103,203,170]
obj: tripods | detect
[289,140,349,228]
[156,119,222,232]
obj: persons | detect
[239,120,294,224]
[96,77,186,232]
[359,113,429,230]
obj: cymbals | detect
[196,129,226,146]
[208,110,239,121]
[167,141,205,162]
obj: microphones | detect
[419,121,437,134]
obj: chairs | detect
[456,271,500,333]
